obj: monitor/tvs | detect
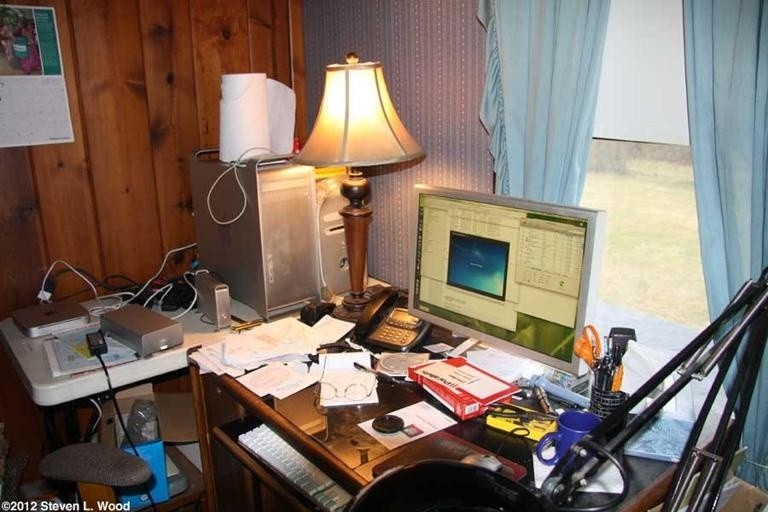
[407,184,603,378]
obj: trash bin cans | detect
[110,414,169,508]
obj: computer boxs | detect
[317,173,369,297]
[191,148,322,321]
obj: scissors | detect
[573,324,601,369]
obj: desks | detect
[186,321,737,512]
[0,274,391,512]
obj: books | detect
[51,319,130,361]
[370,428,529,484]
[620,411,695,464]
[355,399,459,453]
[229,157,286,169]
[440,361,522,408]
[41,339,139,379]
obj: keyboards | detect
[237,424,352,511]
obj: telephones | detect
[355,288,433,351]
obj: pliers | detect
[489,402,555,425]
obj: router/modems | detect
[12,300,90,335]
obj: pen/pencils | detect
[354,363,397,384]
[593,356,625,390]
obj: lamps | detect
[292,52,426,322]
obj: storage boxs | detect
[113,383,199,512]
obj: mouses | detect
[462,452,503,472]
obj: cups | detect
[538,410,601,468]
[589,384,625,435]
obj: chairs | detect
[37,442,152,512]
[342,459,547,512]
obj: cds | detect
[381,351,425,372]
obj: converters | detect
[86,330,108,354]
